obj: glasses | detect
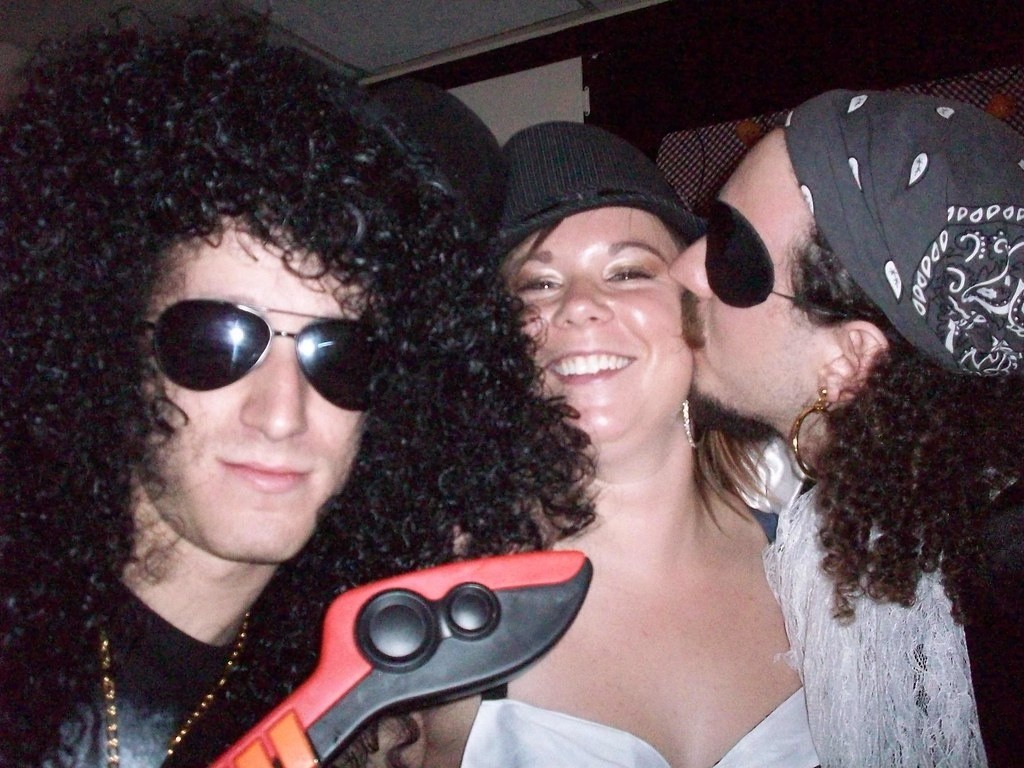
[135,299,377,413]
[704,189,847,323]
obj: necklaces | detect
[99,611,249,768]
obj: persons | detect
[670,90,1024,768]
[370,121,821,768]
[0,1,603,768]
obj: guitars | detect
[206,546,595,768]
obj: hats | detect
[484,120,701,246]
[786,87,1024,381]
